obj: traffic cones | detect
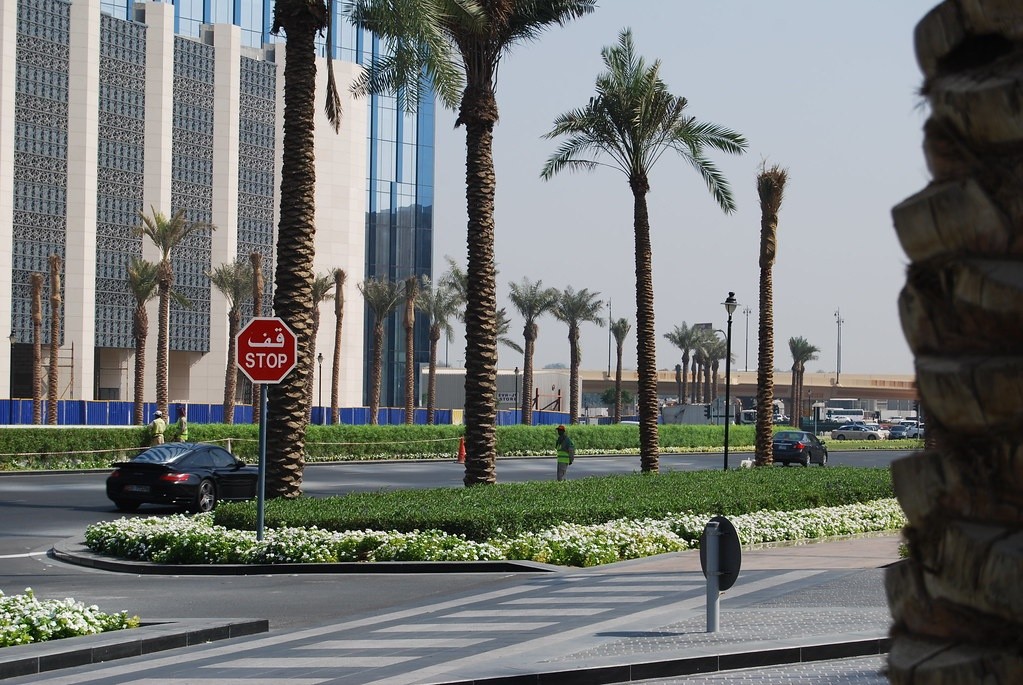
[453,437,466,464]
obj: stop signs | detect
[234,317,298,384]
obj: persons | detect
[177,409,188,442]
[556,425,574,481]
[148,411,166,446]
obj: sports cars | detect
[107,442,259,514]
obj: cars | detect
[831,425,886,441]
[864,410,922,439]
[907,422,925,438]
[887,425,908,440]
[740,409,790,425]
[771,430,829,468]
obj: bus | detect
[825,408,866,423]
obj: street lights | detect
[834,307,845,384]
[606,297,612,380]
[807,388,813,426]
[514,366,520,426]
[743,304,753,372]
[317,352,323,426]
[720,291,741,473]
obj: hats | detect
[556,425,565,431]
[153,411,162,416]
